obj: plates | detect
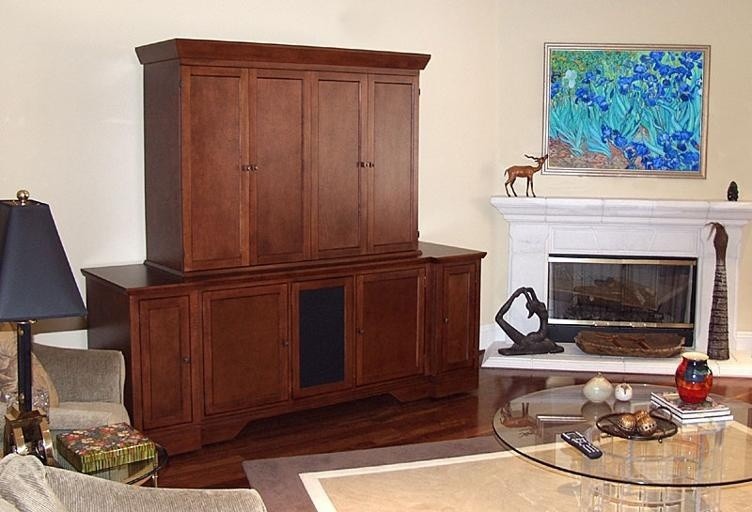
[354,260,428,404]
[311,65,420,259]
[80,264,202,457]
[290,271,358,412]
[143,59,310,276]
[195,271,290,444]
[416,240,490,401]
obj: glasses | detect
[0,189,88,412]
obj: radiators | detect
[561,430,603,460]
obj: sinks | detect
[29,337,131,438]
[0,452,270,512]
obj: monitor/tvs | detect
[650,390,734,425]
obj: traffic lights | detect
[0,321,60,442]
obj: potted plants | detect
[541,43,712,180]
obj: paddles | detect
[240,418,750,511]
